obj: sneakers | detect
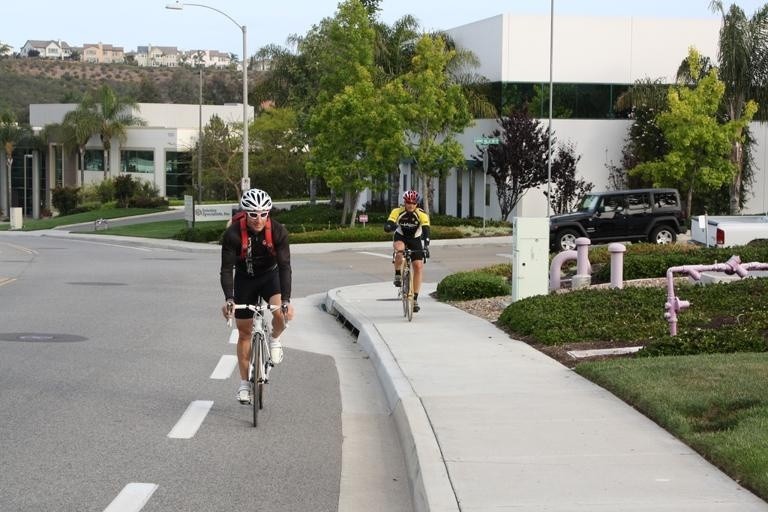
[394,275,401,287]
[413,302,420,311]
[236,386,253,401]
[269,334,283,364]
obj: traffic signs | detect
[473,137,499,146]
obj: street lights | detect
[165,1,251,194]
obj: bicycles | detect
[391,248,428,323]
[225,301,288,428]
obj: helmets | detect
[403,191,422,204]
[241,189,272,211]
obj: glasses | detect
[247,211,268,219]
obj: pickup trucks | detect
[690,213,767,249]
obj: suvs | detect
[549,188,687,255]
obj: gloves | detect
[390,222,399,231]
[424,248,429,258]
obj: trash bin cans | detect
[10,207,22,230]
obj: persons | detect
[383,190,431,311]
[219,189,292,403]
